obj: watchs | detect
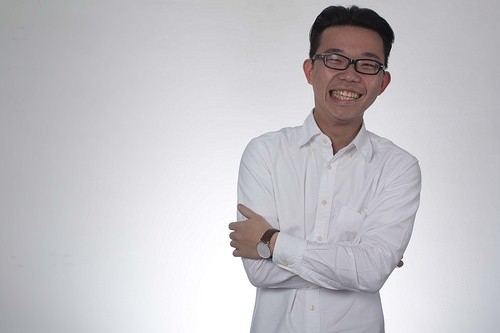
[256,228,280,260]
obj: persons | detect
[229,5,422,333]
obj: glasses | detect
[311,53,385,75]
